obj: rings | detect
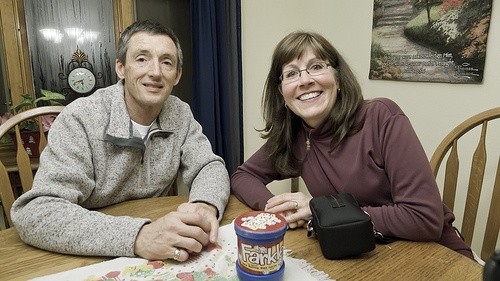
[174,248,181,260]
[293,201,298,209]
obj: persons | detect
[9,18,230,263]
[231,30,486,267]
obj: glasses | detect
[278,61,333,85]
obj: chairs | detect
[429,108,500,265]
[0,105,66,231]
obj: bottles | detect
[233,210,287,281]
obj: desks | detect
[0,194,485,281]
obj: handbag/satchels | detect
[309,190,376,260]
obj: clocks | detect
[67,66,97,95]
